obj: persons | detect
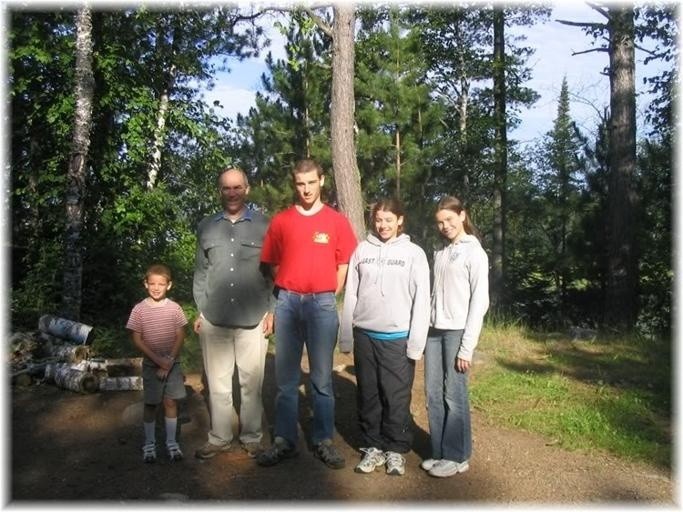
[125,263,189,463]
[420,195,489,479]
[339,198,432,477]
[255,159,358,468]
[193,166,278,460]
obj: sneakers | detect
[165,440,184,462]
[239,440,265,458]
[142,442,156,463]
[355,446,386,475]
[195,439,232,459]
[314,439,346,470]
[421,457,469,478]
[384,450,406,476]
[257,437,294,468]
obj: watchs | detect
[167,355,176,362]
